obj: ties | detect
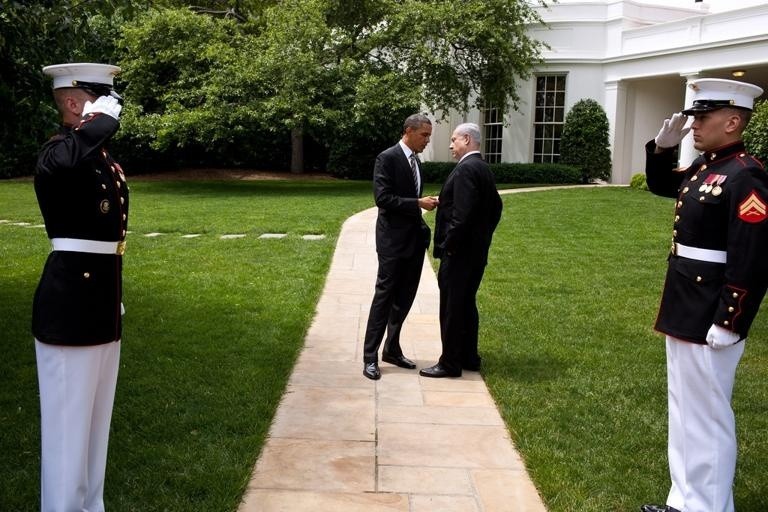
[409,154,419,198]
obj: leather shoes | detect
[641,503,681,512]
[419,363,462,377]
[462,361,480,371]
[382,353,416,369]
[363,361,381,380]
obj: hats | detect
[40,62,125,104]
[682,77,764,115]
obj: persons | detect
[419,121,503,379]
[637,77,768,512]
[29,62,132,512]
[362,113,440,381]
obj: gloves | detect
[706,324,740,351]
[81,93,124,121]
[654,111,692,150]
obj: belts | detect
[670,241,728,264]
[49,237,126,256]
